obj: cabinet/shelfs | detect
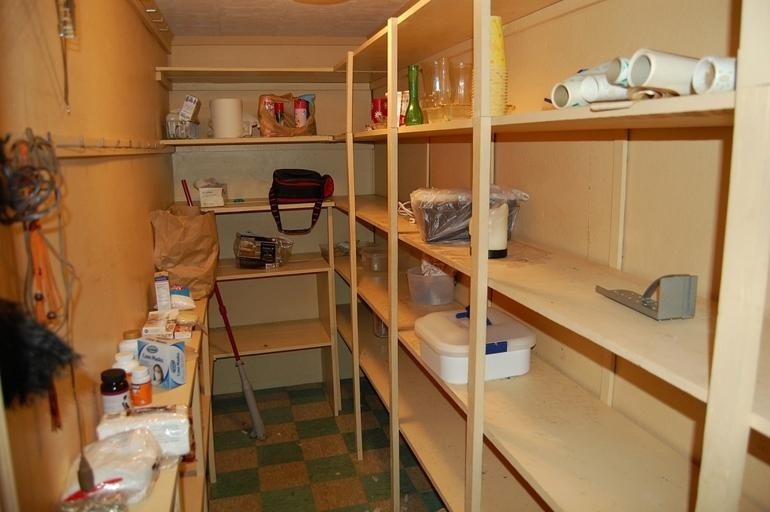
[58,210,225,512]
[145,59,345,423]
[320,0,770,512]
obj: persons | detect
[151,363,164,386]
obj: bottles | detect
[260,98,310,135]
[404,57,471,125]
[99,329,151,415]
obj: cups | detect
[472,15,507,117]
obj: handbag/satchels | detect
[268,168,335,237]
